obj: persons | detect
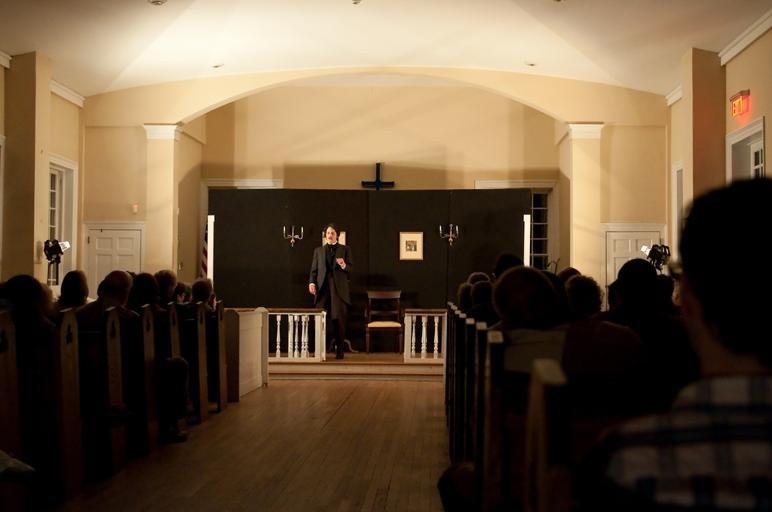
[1,271,219,507]
[305,223,353,363]
[434,173,772,512]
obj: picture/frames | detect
[321,230,346,247]
[400,231,423,261]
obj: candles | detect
[439,224,458,234]
[283,224,304,233]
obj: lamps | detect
[640,244,670,275]
[44,239,71,284]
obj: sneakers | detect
[336,352,343,359]
[169,428,193,442]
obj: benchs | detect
[445,303,571,510]
[0,300,228,512]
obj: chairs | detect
[364,288,405,354]
